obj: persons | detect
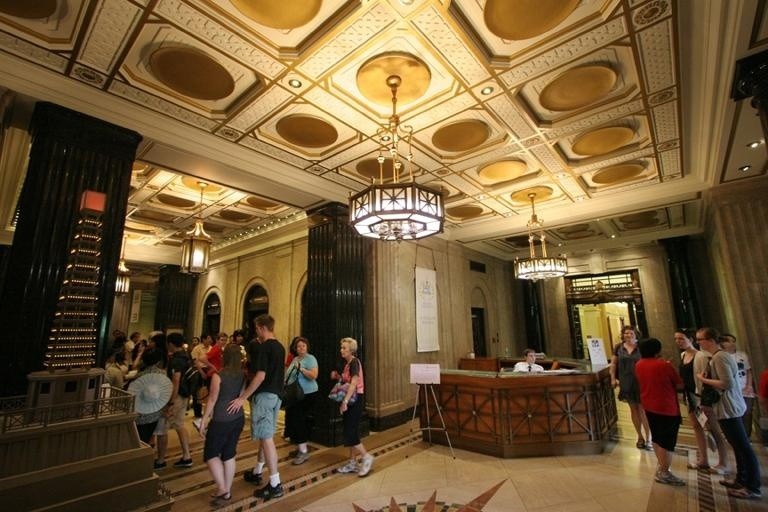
[329,337,375,476]
[609,325,654,451]
[634,338,686,487]
[695,327,762,499]
[673,328,732,474]
[716,334,755,446]
[758,367,768,456]
[512,349,544,373]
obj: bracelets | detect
[342,400,349,404]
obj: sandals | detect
[207,490,232,504]
[687,461,732,475]
[718,474,762,501]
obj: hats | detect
[126,373,175,415]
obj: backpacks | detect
[177,352,204,398]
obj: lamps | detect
[179,182,215,277]
[115,234,133,298]
[348,72,449,248]
[512,190,569,284]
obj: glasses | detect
[695,338,711,341]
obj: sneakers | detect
[253,482,285,499]
[637,437,655,452]
[243,466,264,485]
[357,453,374,478]
[193,417,205,434]
[173,457,194,467]
[336,459,360,474]
[153,461,168,470]
[653,467,686,487]
[287,448,310,465]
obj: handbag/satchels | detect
[700,364,721,406]
[328,381,359,404]
[281,383,305,409]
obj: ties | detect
[527,366,533,372]
